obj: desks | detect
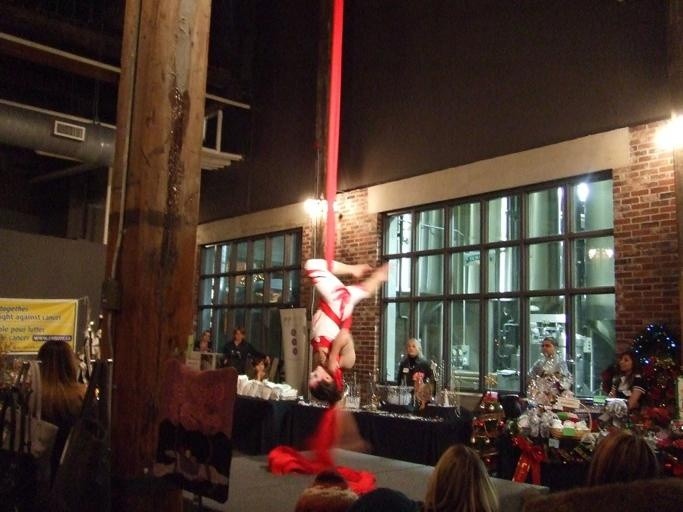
[233,391,469,462]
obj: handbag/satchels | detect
[0,360,59,462]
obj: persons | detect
[222,325,272,377]
[394,337,437,405]
[312,469,349,490]
[27,338,94,483]
[294,482,358,512]
[346,486,420,511]
[422,442,500,511]
[524,430,682,511]
[242,357,270,381]
[526,336,575,396]
[191,329,212,354]
[302,257,390,403]
[603,349,647,413]
